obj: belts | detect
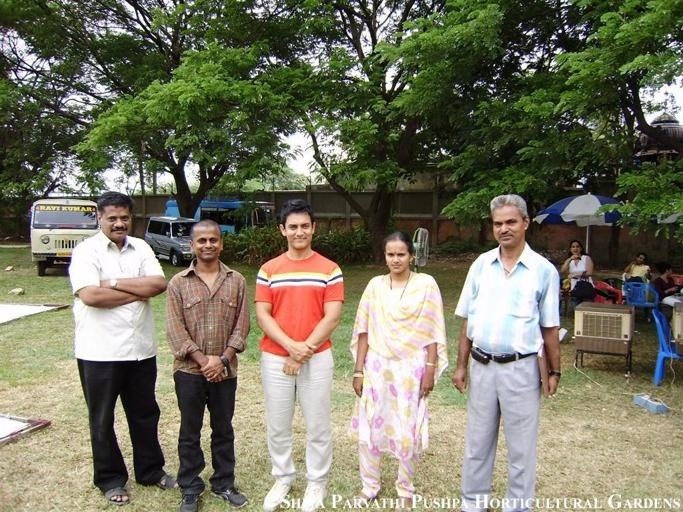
[469,346,538,363]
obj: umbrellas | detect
[532,192,637,253]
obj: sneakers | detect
[261,474,293,512]
[210,488,248,509]
[301,482,326,512]
[180,493,200,512]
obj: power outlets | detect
[624,282,660,308]
[633,392,668,414]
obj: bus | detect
[165,201,275,236]
[30,199,100,276]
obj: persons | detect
[253,199,345,512]
[452,194,561,512]
[349,232,450,512]
[654,261,681,302]
[68,191,180,505]
[561,241,595,317]
[167,219,250,512]
[624,253,651,294]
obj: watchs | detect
[108,277,116,288]
[219,354,230,368]
[550,370,562,377]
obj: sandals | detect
[105,489,130,506]
[154,472,179,492]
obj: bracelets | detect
[352,373,363,377]
[425,362,438,366]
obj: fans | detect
[411,226,429,273]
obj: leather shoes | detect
[395,493,415,512]
[349,490,379,508]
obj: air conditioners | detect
[574,301,635,340]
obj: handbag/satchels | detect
[572,271,594,300]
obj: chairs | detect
[595,281,622,304]
[671,274,683,288]
[601,277,630,303]
[651,307,683,388]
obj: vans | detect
[145,217,197,266]
